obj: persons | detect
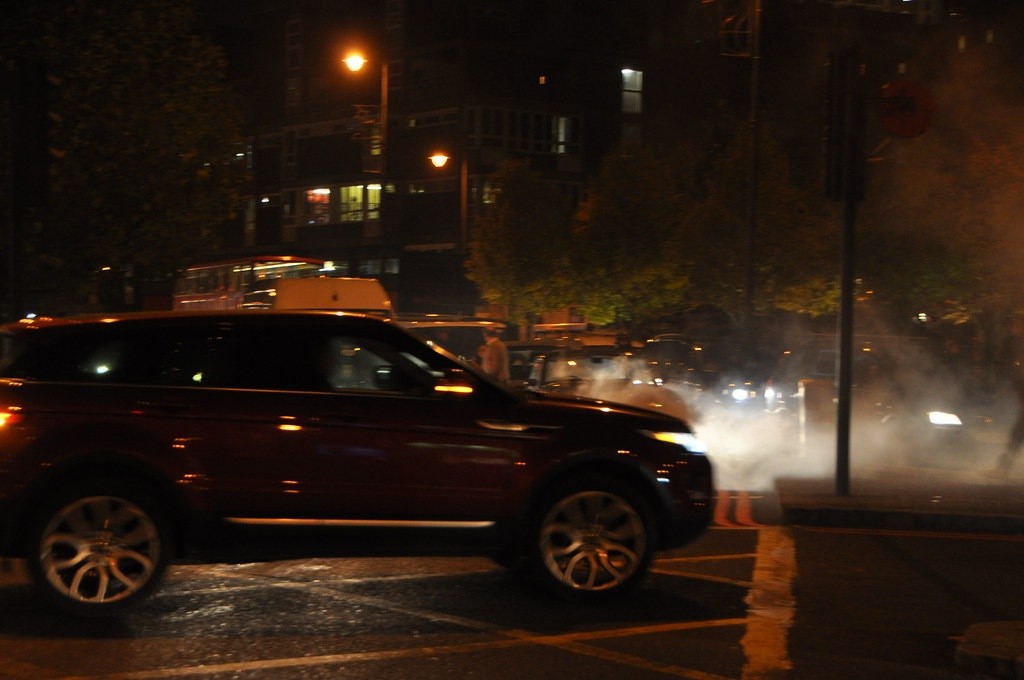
[475,321,511,382]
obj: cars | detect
[644,330,782,408]
[523,346,688,420]
[766,347,1012,462]
[508,340,587,380]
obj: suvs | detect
[1,310,720,616]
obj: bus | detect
[170,256,342,312]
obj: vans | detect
[242,276,400,389]
[389,317,506,379]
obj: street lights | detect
[429,143,467,316]
[339,42,390,289]
